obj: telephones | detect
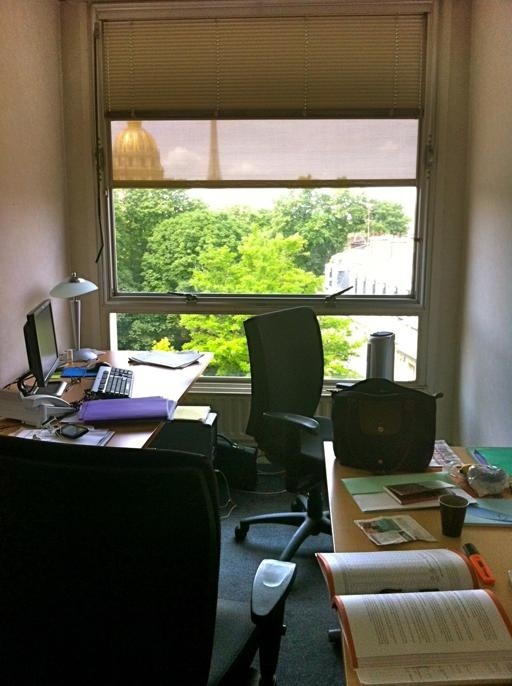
[1,390,75,427]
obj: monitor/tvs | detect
[16,298,68,397]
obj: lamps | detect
[48,271,99,361]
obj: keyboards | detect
[90,365,135,398]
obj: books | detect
[312,548,511,685]
[170,403,214,424]
[383,479,456,506]
[77,395,176,428]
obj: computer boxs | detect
[150,405,218,474]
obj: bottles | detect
[66,349,74,363]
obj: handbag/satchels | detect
[332,376,443,473]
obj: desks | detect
[323,438,512,686]
[0,350,215,451]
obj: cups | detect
[439,494,468,538]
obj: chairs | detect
[0,435,297,684]
[234,307,332,560]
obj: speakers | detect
[366,330,396,382]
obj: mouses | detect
[94,361,111,371]
